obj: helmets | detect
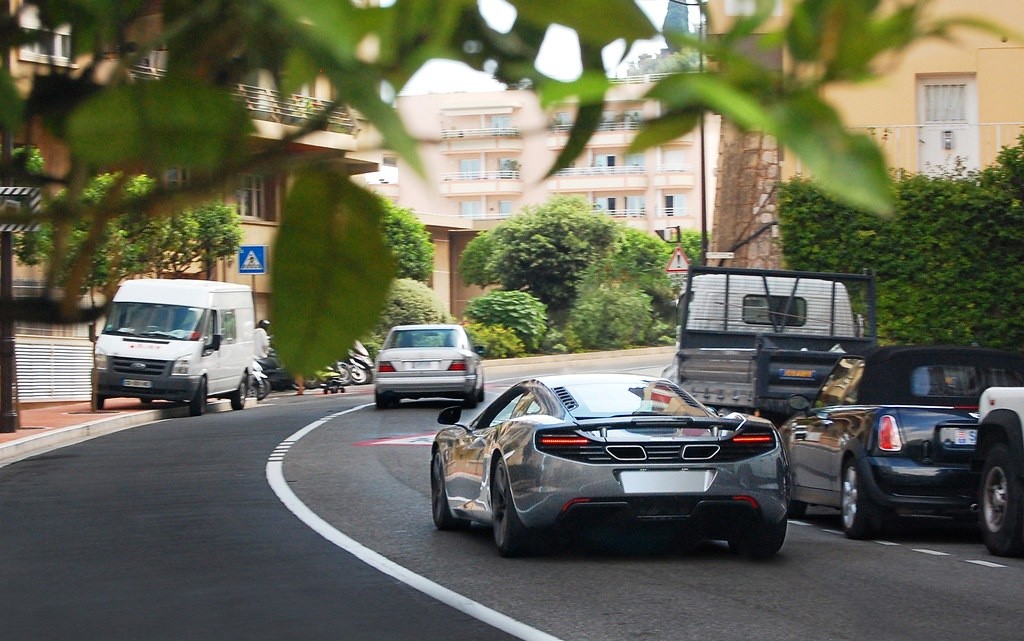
[259,320,270,329]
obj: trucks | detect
[655,257,1019,448]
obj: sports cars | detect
[432,374,791,569]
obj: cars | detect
[374,325,485,409]
[777,343,1024,541]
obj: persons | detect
[254,319,270,360]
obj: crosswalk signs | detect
[238,245,266,275]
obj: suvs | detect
[973,385,1024,556]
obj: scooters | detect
[339,330,373,384]
[303,355,355,392]
[246,336,288,401]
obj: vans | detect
[88,278,255,416]
